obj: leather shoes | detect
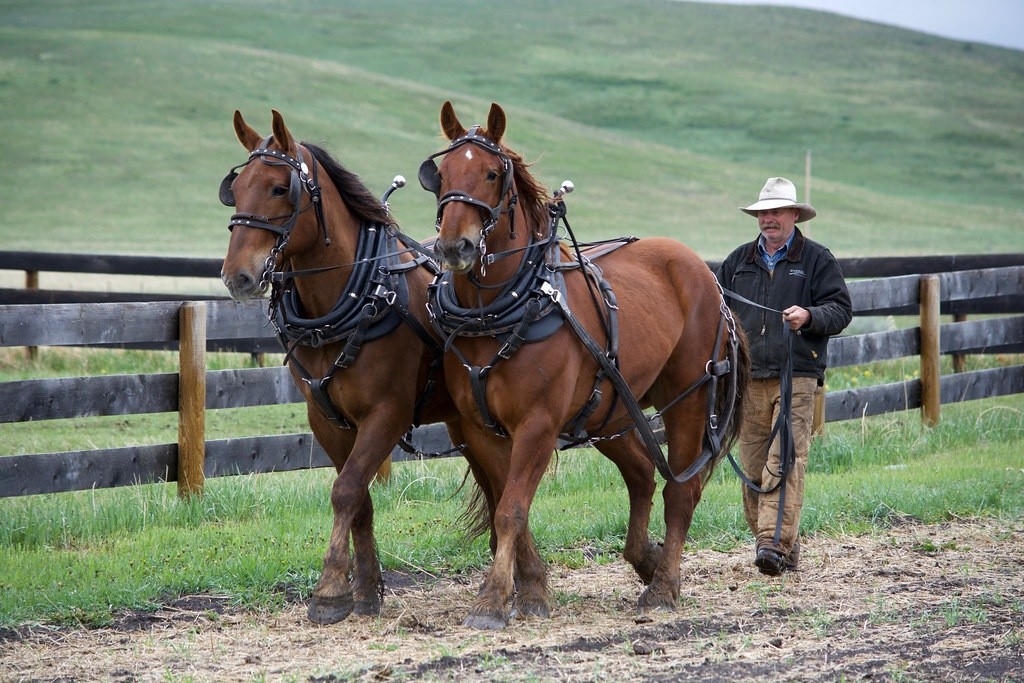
[754,548,799,576]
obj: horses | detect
[433,101,752,631]
[218,108,499,623]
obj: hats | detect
[738,177,817,224]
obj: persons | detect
[715,177,852,576]
